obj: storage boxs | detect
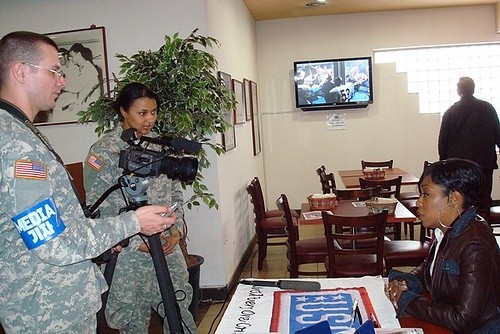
[363,170,385,179]
[365,201,398,215]
[307,195,337,211]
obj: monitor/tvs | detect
[293,56,373,108]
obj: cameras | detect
[158,202,178,217]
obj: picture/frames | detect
[218,71,261,156]
[33,26,111,126]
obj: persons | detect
[0,31,175,334]
[60,43,101,111]
[83,82,197,334]
[438,77,500,232]
[296,65,368,105]
[52,48,79,115]
[387,158,500,334]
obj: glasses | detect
[25,61,65,82]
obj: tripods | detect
[84,174,183,334]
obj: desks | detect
[300,199,416,240]
[399,289,453,334]
[365,209,366,209]
[338,169,420,200]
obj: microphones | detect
[241,280,320,291]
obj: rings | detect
[164,224,167,230]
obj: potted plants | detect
[76,28,239,322]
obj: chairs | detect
[245,159,435,279]
